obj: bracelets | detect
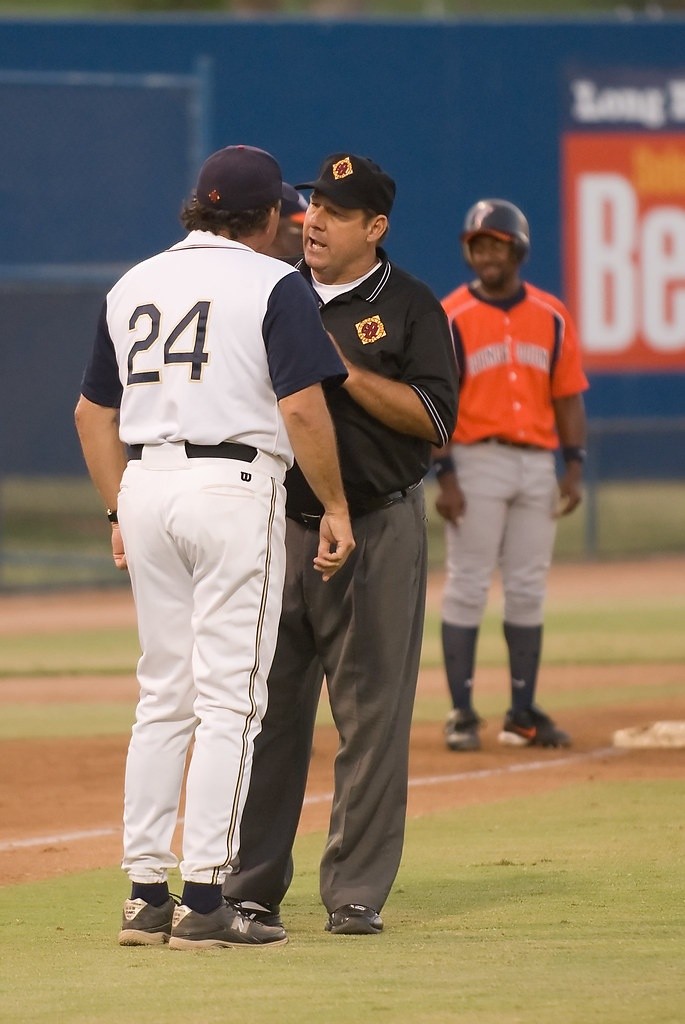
[106,509,118,524]
[435,454,455,479]
[564,444,587,463]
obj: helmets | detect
[461,198,531,267]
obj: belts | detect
[127,441,258,464]
[483,435,545,451]
[286,479,423,526]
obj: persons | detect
[222,152,461,933]
[431,198,590,752]
[74,145,355,951]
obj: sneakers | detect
[223,897,283,929]
[499,708,569,748]
[324,904,384,934]
[443,706,488,749]
[169,898,287,950]
[118,892,182,945]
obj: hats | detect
[295,152,396,218]
[197,145,299,208]
